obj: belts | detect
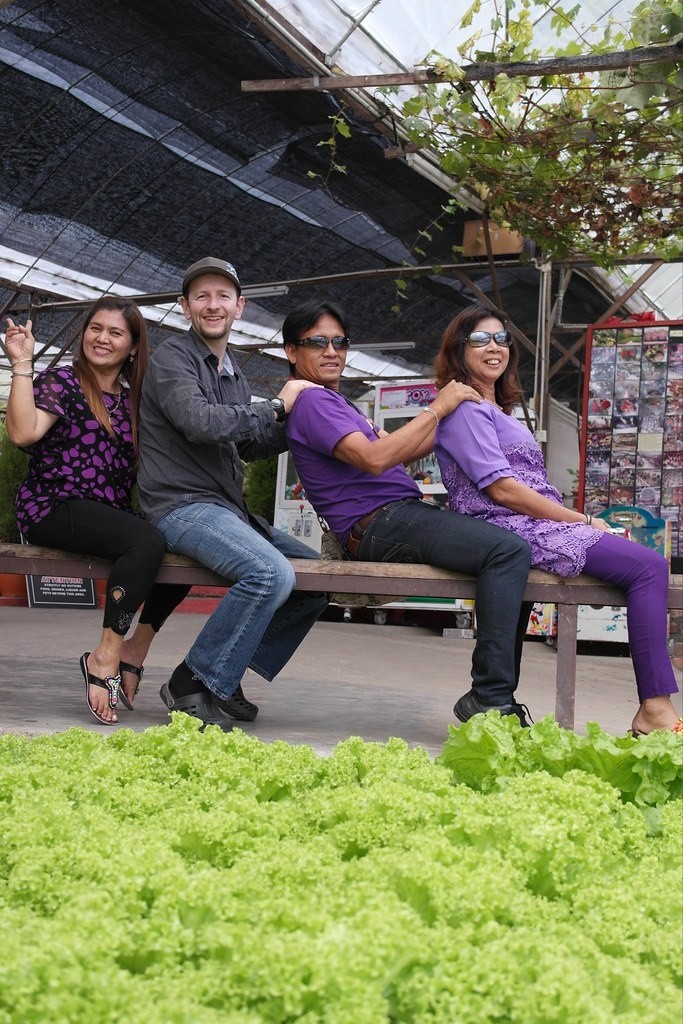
[351,509,377,540]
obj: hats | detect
[182,257,241,296]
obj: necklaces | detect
[104,383,120,423]
[484,397,503,411]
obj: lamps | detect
[404,151,486,215]
[242,285,290,298]
[348,341,416,351]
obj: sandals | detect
[160,677,233,732]
[214,681,258,720]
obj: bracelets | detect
[585,513,592,525]
[10,359,33,378]
[422,407,439,425]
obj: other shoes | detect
[454,688,534,727]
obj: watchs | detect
[269,397,285,422]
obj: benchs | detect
[0,539,683,739]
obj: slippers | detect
[116,659,143,710]
[632,719,683,739]
[80,652,118,725]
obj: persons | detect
[433,304,683,741]
[282,296,533,729]
[138,258,332,733]
[5,297,192,726]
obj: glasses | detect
[464,330,513,347]
[291,336,350,351]
